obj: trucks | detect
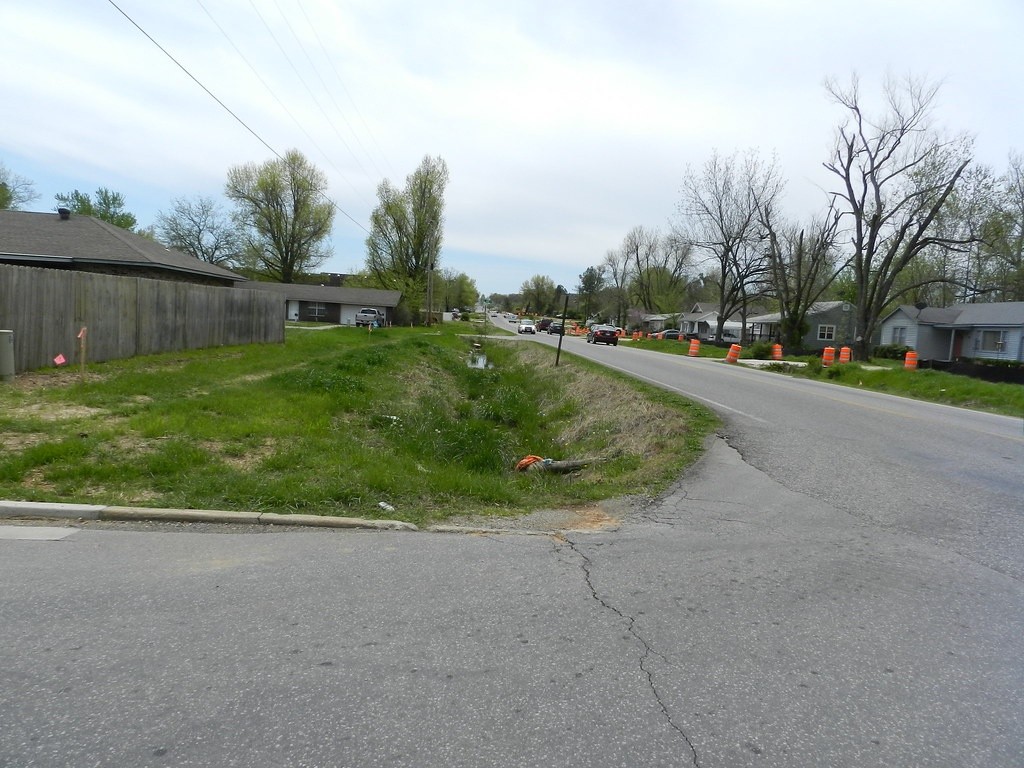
[535,318,553,332]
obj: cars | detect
[491,311,499,317]
[517,319,537,334]
[547,321,565,335]
[504,313,518,323]
[586,324,619,346]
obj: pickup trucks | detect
[356,308,385,329]
